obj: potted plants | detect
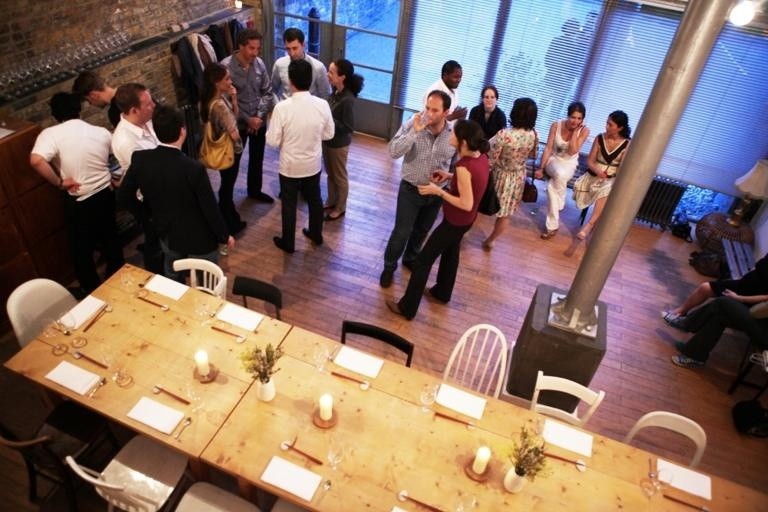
[241,342,285,402]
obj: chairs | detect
[271,498,309,512]
[0,401,90,504]
[65,434,188,512]
[232,276,282,324]
[7,277,78,348]
[173,259,226,300]
[443,324,507,399]
[175,482,261,512]
[341,320,414,367]
[531,370,606,428]
[625,411,707,468]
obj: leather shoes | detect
[232,221,246,233]
[403,258,416,268]
[303,229,322,244]
[253,192,274,203]
[380,267,392,287]
[274,236,294,253]
[68,287,89,298]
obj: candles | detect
[320,393,333,420]
[472,446,491,475]
[194,350,210,376]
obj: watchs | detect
[57,177,63,187]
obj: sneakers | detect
[661,312,688,331]
[671,355,704,370]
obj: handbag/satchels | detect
[476,154,500,215]
[733,399,768,437]
[689,251,724,279]
[750,349,768,374]
[522,130,537,202]
[199,99,237,170]
[669,212,694,243]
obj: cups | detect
[653,471,673,499]
[194,295,209,329]
[120,271,139,304]
[103,354,133,386]
[313,343,329,375]
[420,386,436,414]
[40,319,67,355]
[419,111,432,126]
[186,381,203,415]
[417,175,429,187]
[57,313,85,348]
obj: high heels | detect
[482,236,495,251]
[424,287,451,304]
[386,299,415,321]
[576,224,589,240]
[541,227,557,239]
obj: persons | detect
[666,252,768,307]
[537,18,579,127]
[380,91,460,289]
[383,118,490,320]
[120,102,235,283]
[482,98,538,251]
[574,12,599,70]
[661,297,768,368]
[114,82,164,170]
[219,29,276,205]
[573,110,633,240]
[470,86,506,141]
[271,27,333,108]
[73,69,158,127]
[197,64,245,255]
[266,59,335,255]
[321,58,365,220]
[536,102,589,239]
[421,60,468,124]
[30,91,123,292]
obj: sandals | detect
[325,209,346,221]
[322,203,336,210]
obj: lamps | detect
[726,159,768,227]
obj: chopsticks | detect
[156,385,190,406]
[435,412,473,426]
[211,327,243,339]
[284,443,322,465]
[402,496,443,512]
[83,305,108,332]
[544,452,584,466]
[664,494,710,512]
[139,297,164,308]
[78,352,108,369]
[332,371,366,384]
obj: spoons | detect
[327,344,339,359]
[139,275,152,287]
[175,418,191,438]
[62,328,70,335]
[316,480,331,505]
[88,378,105,399]
[211,302,224,317]
[649,457,655,479]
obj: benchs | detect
[722,238,768,396]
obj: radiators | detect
[636,176,688,232]
[526,141,610,226]
